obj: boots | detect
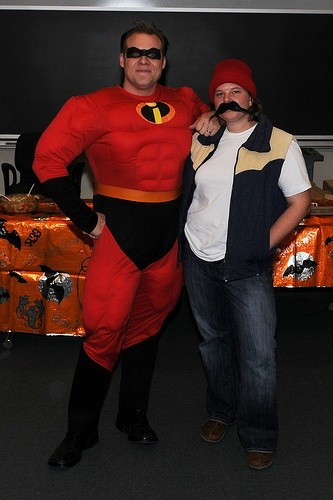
[115,331,161,444]
[48,343,112,469]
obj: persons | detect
[32,22,221,471]
[180,58,314,469]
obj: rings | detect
[207,130,212,134]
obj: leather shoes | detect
[200,419,226,444]
[246,450,274,468]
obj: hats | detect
[209,59,256,103]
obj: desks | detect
[0,214,333,353]
